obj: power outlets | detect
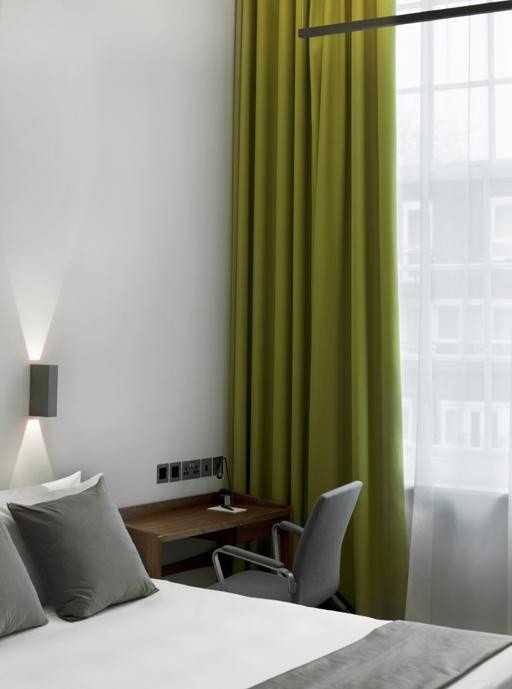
[182,461,191,480]
[191,460,200,479]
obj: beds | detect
[0,469,511,687]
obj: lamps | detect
[29,364,57,418]
[217,456,237,506]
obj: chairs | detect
[206,480,363,612]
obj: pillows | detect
[0,520,49,638]
[0,470,82,500]
[0,473,104,509]
[7,476,159,623]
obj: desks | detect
[118,488,293,579]
